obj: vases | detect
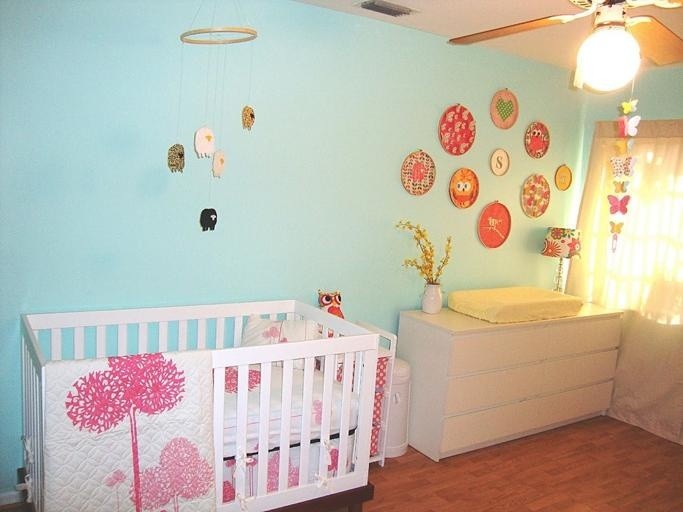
[421,283,442,315]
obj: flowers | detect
[394,219,453,283]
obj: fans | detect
[439,2,683,66]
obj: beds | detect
[15,301,374,512]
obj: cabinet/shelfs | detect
[394,303,626,462]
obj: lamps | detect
[576,7,642,91]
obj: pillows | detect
[233,311,319,375]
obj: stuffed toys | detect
[314,287,345,338]
